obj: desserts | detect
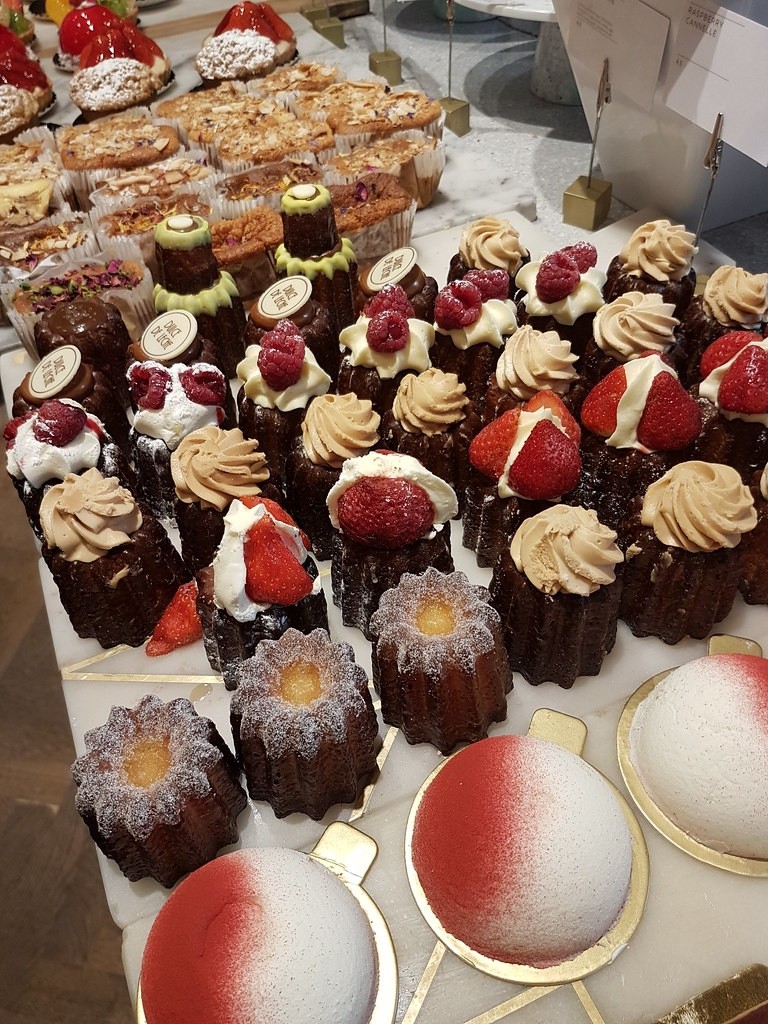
[0,0,767,889]
